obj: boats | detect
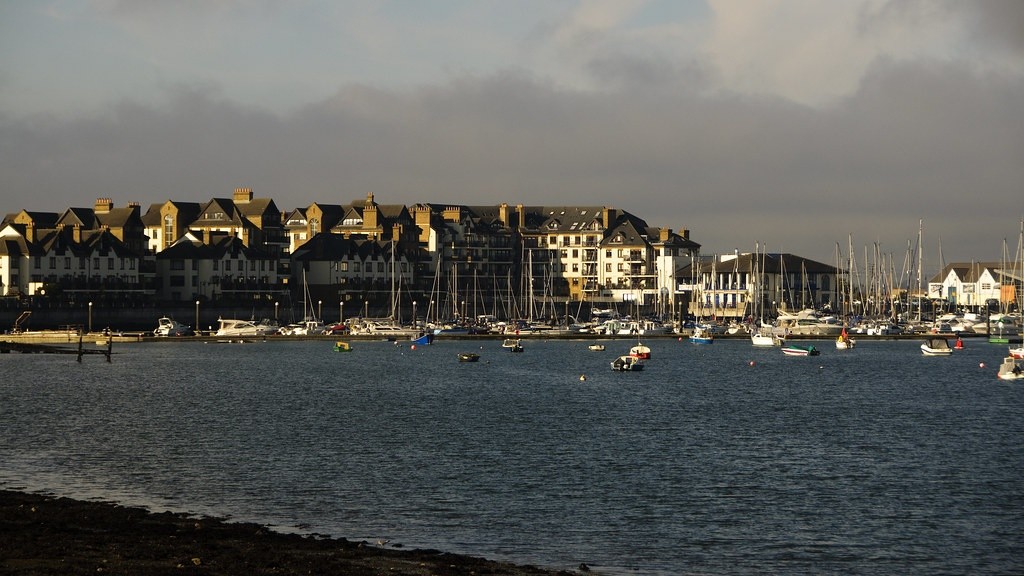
[781,344,819,356]
[153,315,192,336]
[610,355,645,371]
[502,338,524,352]
[332,341,354,352]
[997,355,1024,381]
[459,352,481,362]
[1008,345,1024,359]
[588,343,605,351]
[920,337,953,356]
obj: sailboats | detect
[628,288,652,359]
[215,218,1024,348]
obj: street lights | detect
[318,300,322,320]
[462,301,465,323]
[413,301,417,329]
[275,301,278,326]
[339,301,343,322]
[196,300,200,331]
[431,300,435,324]
[364,301,369,321]
[88,301,93,332]
